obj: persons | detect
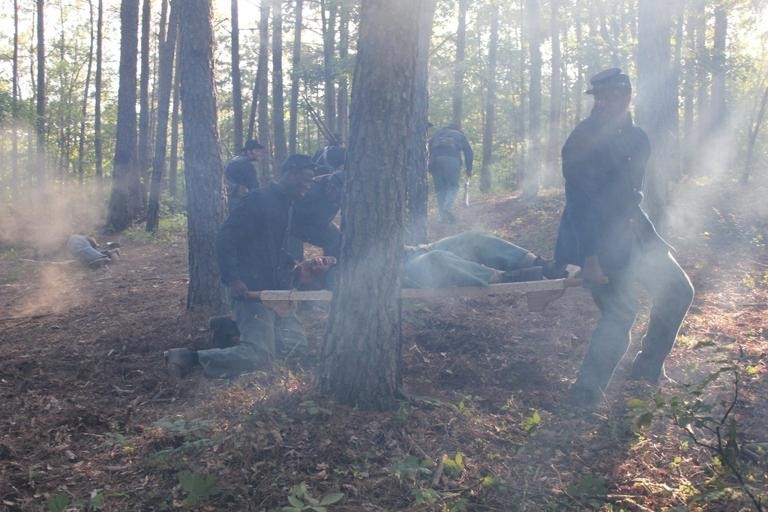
[225,140,265,211]
[428,121,474,223]
[294,165,348,261]
[41,210,114,269]
[309,133,346,175]
[164,153,314,382]
[553,68,696,404]
[291,231,584,290]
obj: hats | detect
[241,139,266,151]
[584,68,631,94]
[282,153,319,174]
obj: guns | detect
[464,179,470,207]
[303,95,338,146]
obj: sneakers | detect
[631,350,683,389]
[500,255,569,283]
[211,315,239,349]
[163,347,194,382]
